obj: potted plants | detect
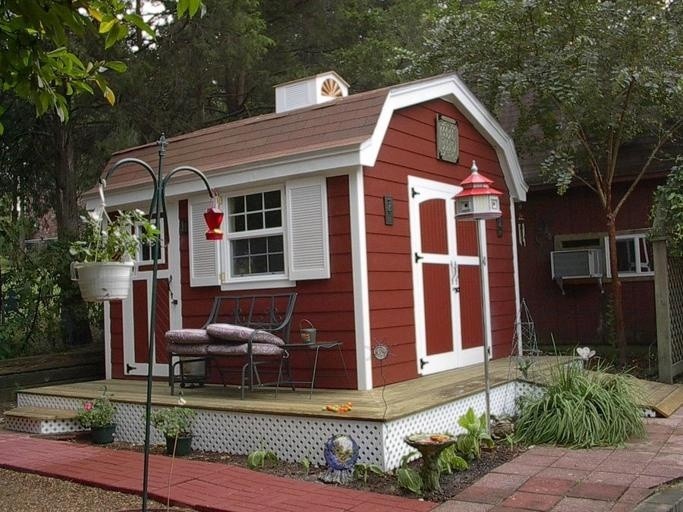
[142,407,197,456]
[70,207,160,303]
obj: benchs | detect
[162,290,299,400]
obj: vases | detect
[92,423,116,444]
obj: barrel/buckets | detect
[299,319,318,345]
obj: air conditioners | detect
[550,249,605,279]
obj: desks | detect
[273,341,352,399]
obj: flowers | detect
[78,384,118,429]
